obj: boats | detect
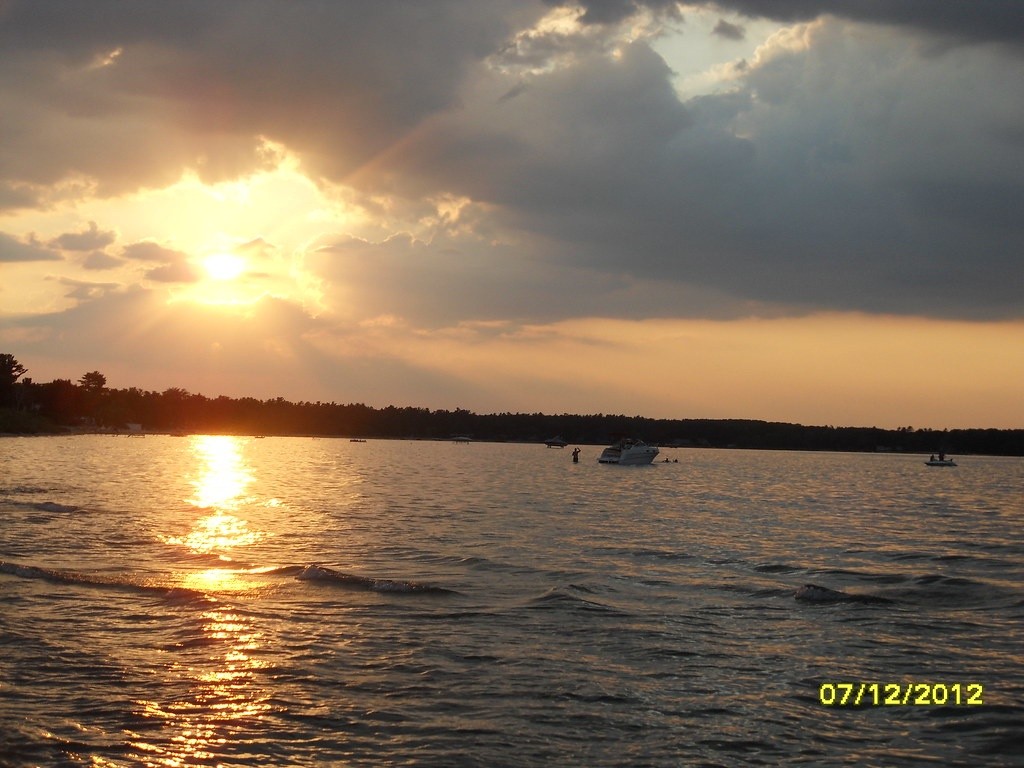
[923,460,958,467]
[597,439,660,466]
[350,440,366,442]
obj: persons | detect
[662,458,669,463]
[673,459,678,462]
[930,452,944,461]
[572,447,581,463]
